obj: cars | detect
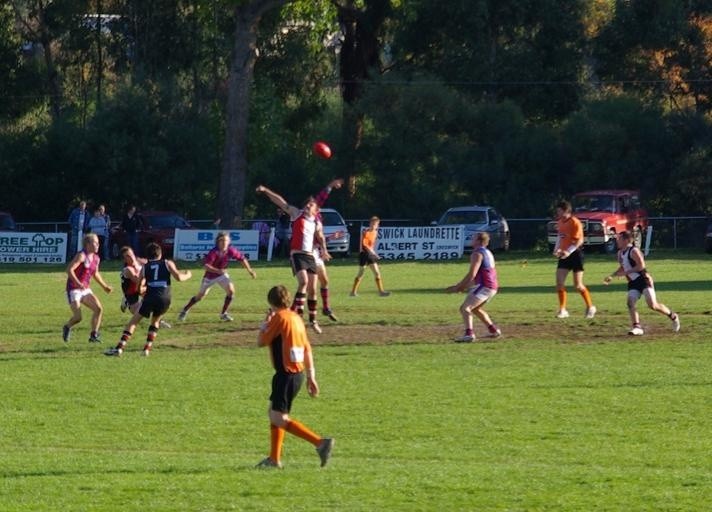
[292,207,351,254]
[434,207,513,251]
[112,212,190,254]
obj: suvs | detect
[551,194,644,250]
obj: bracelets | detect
[305,368,316,379]
[566,243,577,255]
[369,249,375,256]
[259,320,268,330]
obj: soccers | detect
[315,142,330,159]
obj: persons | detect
[271,206,289,259]
[551,200,598,319]
[61,232,113,345]
[0,210,17,232]
[67,199,142,265]
[348,216,390,297]
[209,216,222,230]
[117,244,173,332]
[254,284,335,472]
[253,184,333,334]
[604,196,626,213]
[103,242,192,356]
[176,230,257,323]
[604,230,681,336]
[290,178,348,322]
[443,231,505,342]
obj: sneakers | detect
[316,438,334,467]
[103,348,148,357]
[88,337,104,343]
[454,333,476,343]
[584,305,597,319]
[350,292,391,297]
[121,297,171,330]
[309,309,337,334]
[628,327,644,336]
[62,324,70,342]
[672,313,680,332]
[554,309,569,318]
[178,311,232,322]
[254,457,283,469]
[480,329,501,338]
